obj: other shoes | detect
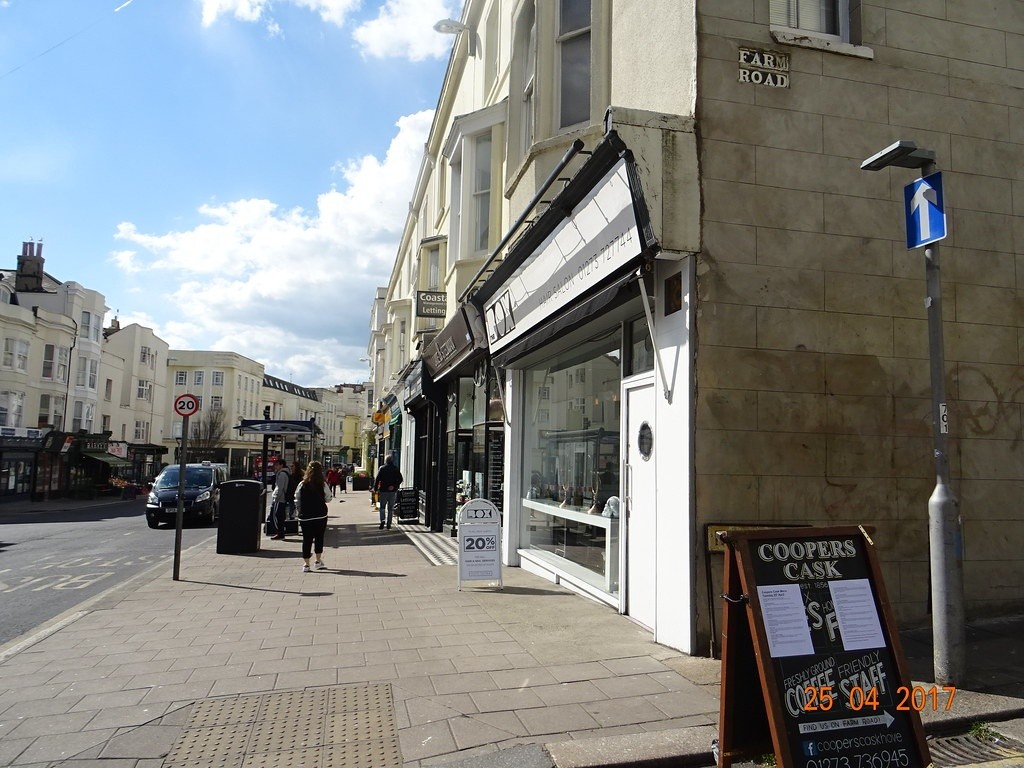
[379,521,385,529]
[271,533,285,540]
[304,564,310,572]
[387,525,391,529]
[314,561,326,569]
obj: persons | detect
[294,460,332,572]
[594,462,618,537]
[374,455,403,530]
[284,460,304,535]
[270,459,291,540]
[327,466,337,499]
[340,468,347,494]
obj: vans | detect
[145,461,226,529]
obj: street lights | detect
[859,140,968,691]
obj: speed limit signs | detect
[174,393,198,417]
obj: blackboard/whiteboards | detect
[716,526,936,768]
[397,487,420,525]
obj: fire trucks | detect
[252,456,278,483]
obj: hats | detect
[278,459,286,465]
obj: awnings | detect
[84,451,133,468]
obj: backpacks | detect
[277,470,298,501]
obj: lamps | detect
[432,19,476,56]
[359,357,371,366]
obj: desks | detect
[522,498,619,593]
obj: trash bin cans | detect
[217,480,266,557]
